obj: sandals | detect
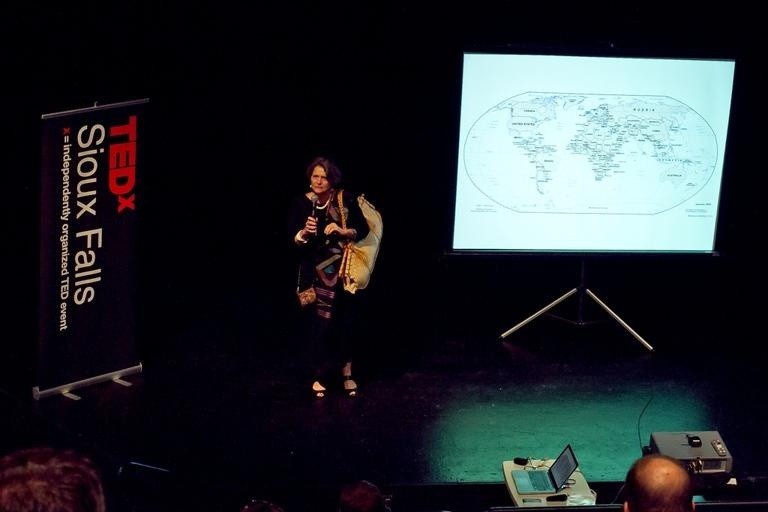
[310,385,327,399]
[342,374,359,397]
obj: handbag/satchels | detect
[296,287,316,307]
[337,190,384,294]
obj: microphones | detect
[311,194,319,218]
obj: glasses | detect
[383,494,393,506]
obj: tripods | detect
[497,257,656,354]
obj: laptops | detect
[511,444,579,495]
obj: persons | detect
[292,157,369,397]
[0,448,106,510]
[337,479,390,512]
[242,496,284,512]
[621,453,696,510]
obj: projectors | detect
[650,430,733,475]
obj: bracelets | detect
[295,230,308,244]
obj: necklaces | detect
[316,194,332,210]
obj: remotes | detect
[711,439,727,456]
